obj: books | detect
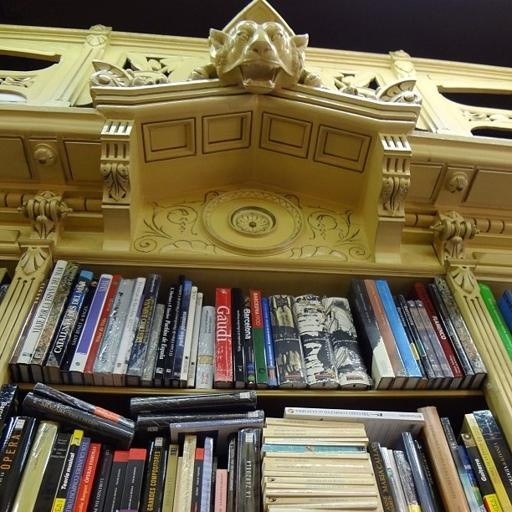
[8,256,490,391]
[0,383,512,512]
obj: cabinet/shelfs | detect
[1,260,512,511]
